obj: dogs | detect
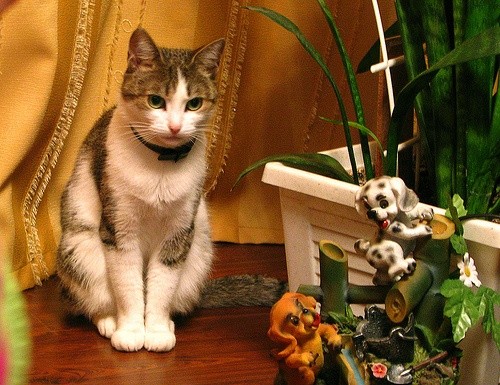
[268,291,345,385]
[353,174,434,290]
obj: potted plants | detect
[230,0,500,385]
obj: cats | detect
[55,25,288,352]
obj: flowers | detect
[442,195,500,350]
[371,363,388,378]
